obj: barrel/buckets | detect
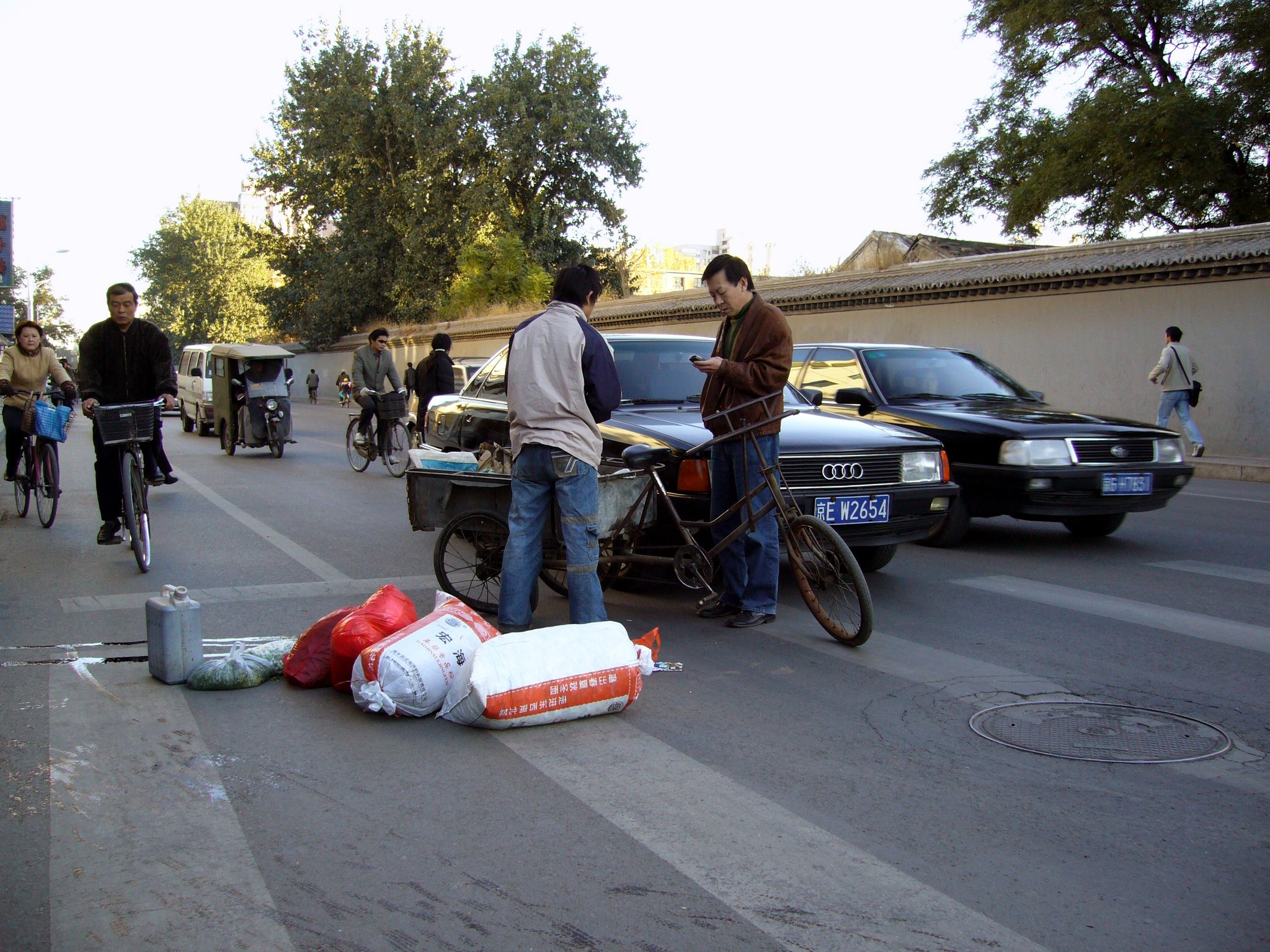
[145,583,204,684]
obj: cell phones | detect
[689,355,706,364]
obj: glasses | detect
[374,340,388,344]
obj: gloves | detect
[0,379,15,398]
[397,387,408,396]
[359,387,370,398]
[61,381,77,402]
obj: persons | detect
[351,327,407,465]
[403,362,416,408]
[47,359,81,411]
[415,334,455,443]
[692,255,792,628]
[0,321,76,494]
[79,283,180,545]
[495,264,621,635]
[306,369,319,399]
[336,371,352,404]
[234,361,263,445]
[1149,326,1205,457]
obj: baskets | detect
[91,399,154,445]
[378,398,406,419]
[21,399,77,443]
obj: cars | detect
[414,328,962,591]
[785,340,1199,552]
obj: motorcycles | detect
[209,343,298,459]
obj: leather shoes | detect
[143,463,165,482]
[695,599,741,617]
[4,465,17,481]
[725,610,777,627]
[47,489,61,496]
[96,520,122,543]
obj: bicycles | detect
[85,396,167,573]
[307,383,318,404]
[48,382,77,421]
[0,388,72,529]
[345,389,414,478]
[336,383,351,409]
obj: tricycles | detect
[402,386,874,648]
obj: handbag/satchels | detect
[1186,381,1201,407]
[21,390,71,442]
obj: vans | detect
[176,343,232,437]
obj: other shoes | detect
[234,439,243,445]
[152,475,179,486]
[382,455,400,465]
[1192,445,1205,456]
[356,433,365,445]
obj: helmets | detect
[344,378,349,382]
